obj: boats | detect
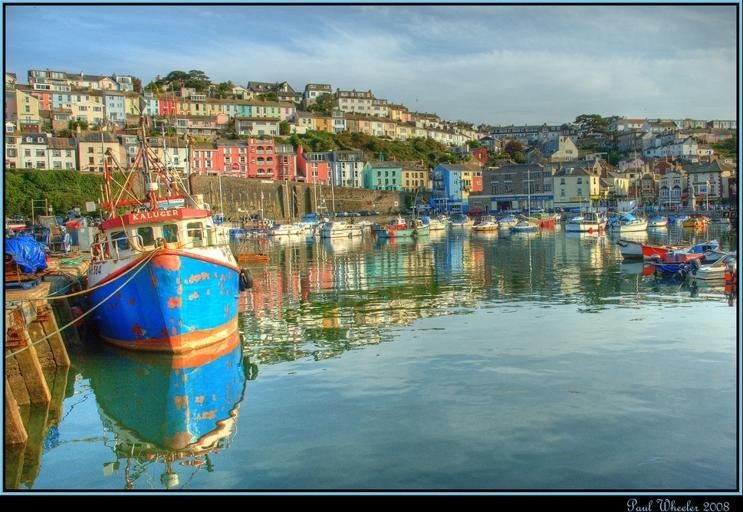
[57,347,262,487]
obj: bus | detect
[4,218,25,230]
